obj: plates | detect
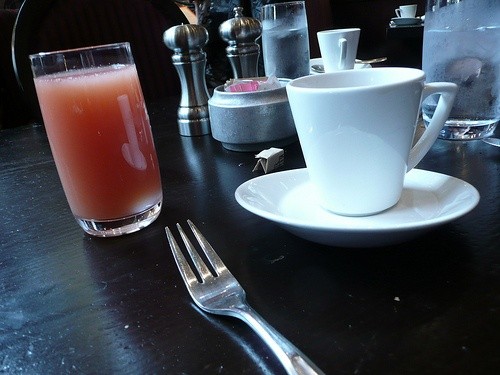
[310,57,372,75]
[234,168,480,248]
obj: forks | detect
[165,218,326,375]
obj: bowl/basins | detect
[391,17,422,25]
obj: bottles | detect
[163,22,210,137]
[218,7,262,79]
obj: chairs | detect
[10,0,196,87]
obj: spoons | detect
[311,57,387,73]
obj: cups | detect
[207,77,297,152]
[286,67,459,217]
[29,42,164,238]
[421,0,500,141]
[395,5,417,17]
[261,1,311,80]
[317,27,361,73]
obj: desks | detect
[0,77,500,375]
